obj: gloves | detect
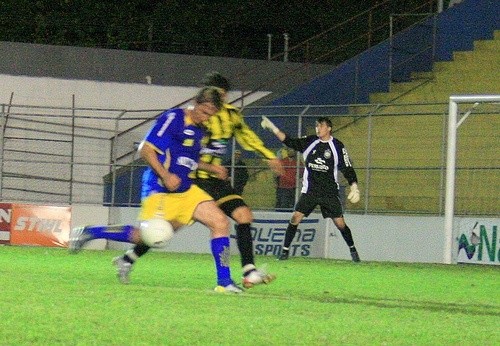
[261,116,274,131]
[347,187,360,202]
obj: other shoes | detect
[352,252,359,262]
[280,254,287,261]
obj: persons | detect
[261,114,361,263]
[112,72,283,289]
[69,86,244,294]
[275,146,303,211]
[223,150,248,196]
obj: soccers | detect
[140,218,173,248]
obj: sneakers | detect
[215,283,244,294]
[71,227,90,252]
[114,256,133,284]
[241,271,275,289]
